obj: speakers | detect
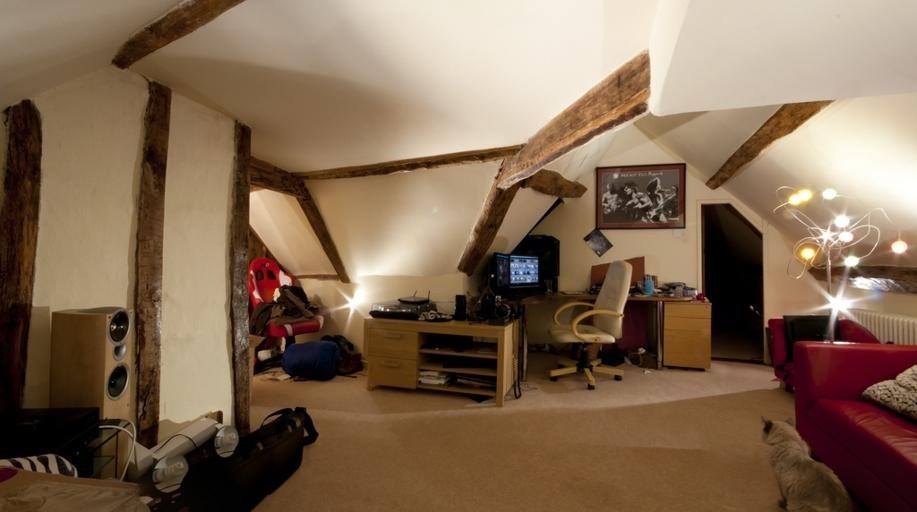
[50,306,132,481]
[455,295,466,321]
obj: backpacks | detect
[280,341,346,383]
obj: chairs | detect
[248,257,324,375]
[768,315,882,392]
[547,260,633,390]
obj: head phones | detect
[493,300,520,319]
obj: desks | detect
[87,419,136,479]
[500,295,712,382]
[1,466,151,512]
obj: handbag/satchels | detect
[178,405,320,512]
[320,334,363,374]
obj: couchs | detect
[791,339,916,511]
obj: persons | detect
[601,175,672,224]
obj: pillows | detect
[861,364,916,423]
[784,315,841,361]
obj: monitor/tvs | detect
[488,253,541,298]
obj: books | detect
[418,369,447,388]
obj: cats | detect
[759,415,856,511]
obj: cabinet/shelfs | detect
[363,315,521,407]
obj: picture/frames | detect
[595,162,686,229]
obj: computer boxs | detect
[512,235,560,279]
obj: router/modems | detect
[398,289,431,305]
